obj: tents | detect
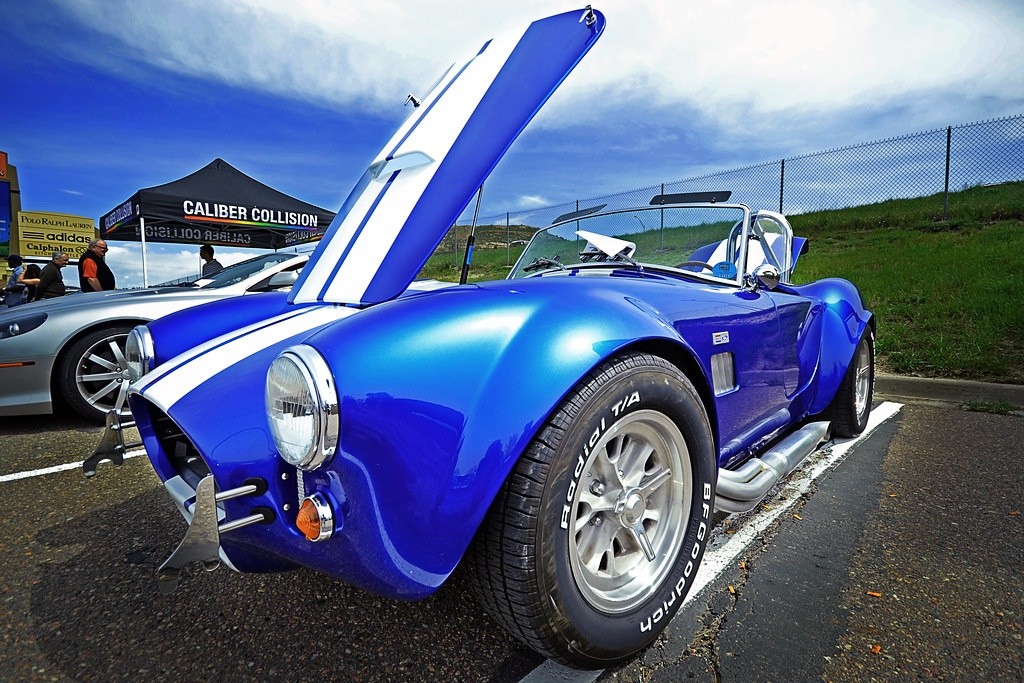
[99,157,336,289]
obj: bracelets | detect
[20,272,24,274]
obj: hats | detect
[4,254,23,262]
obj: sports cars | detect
[122,4,878,671]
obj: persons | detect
[16,264,42,303]
[30,252,70,302]
[78,239,115,293]
[200,244,223,277]
[3,254,29,309]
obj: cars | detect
[0,253,328,424]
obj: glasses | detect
[94,244,109,251]
